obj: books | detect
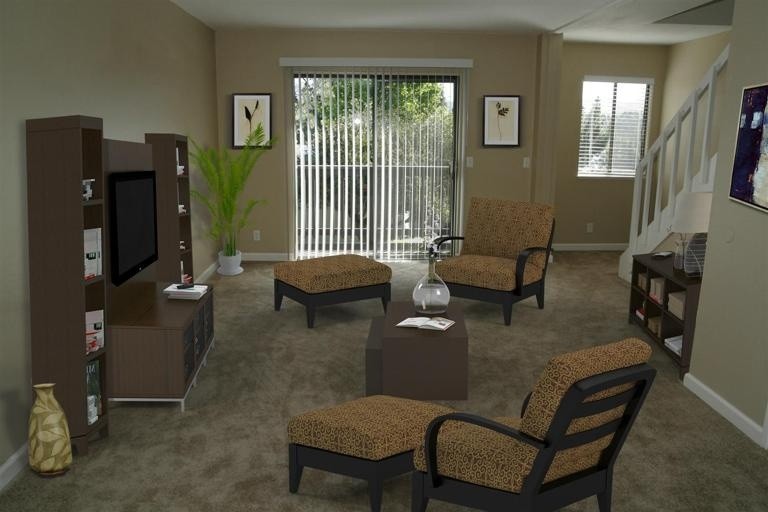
[162,283,208,300]
[664,342,681,357]
[664,335,683,354]
[394,317,455,331]
[635,307,645,321]
[83,227,105,426]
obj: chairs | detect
[431,195,557,326]
[408,338,658,511]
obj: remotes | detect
[178,285,193,289]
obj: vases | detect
[673,240,689,270]
[410,257,449,313]
[29,383,76,479]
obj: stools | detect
[282,394,456,511]
[272,253,392,329]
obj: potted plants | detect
[187,125,274,276]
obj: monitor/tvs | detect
[109,169,158,287]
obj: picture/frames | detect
[230,93,274,149]
[727,83,768,215]
[482,94,521,148]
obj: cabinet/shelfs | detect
[627,251,704,380]
